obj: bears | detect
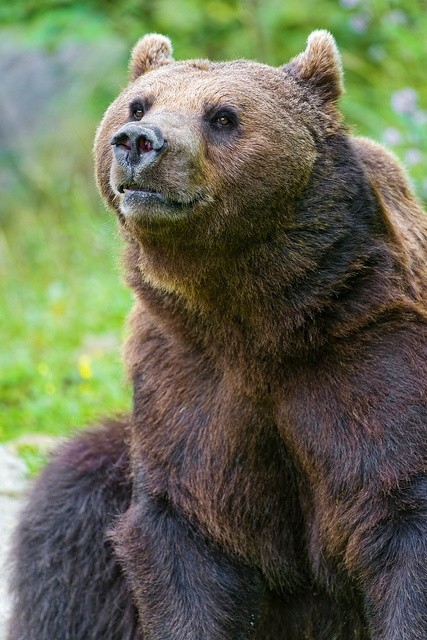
[0,25,427,640]
